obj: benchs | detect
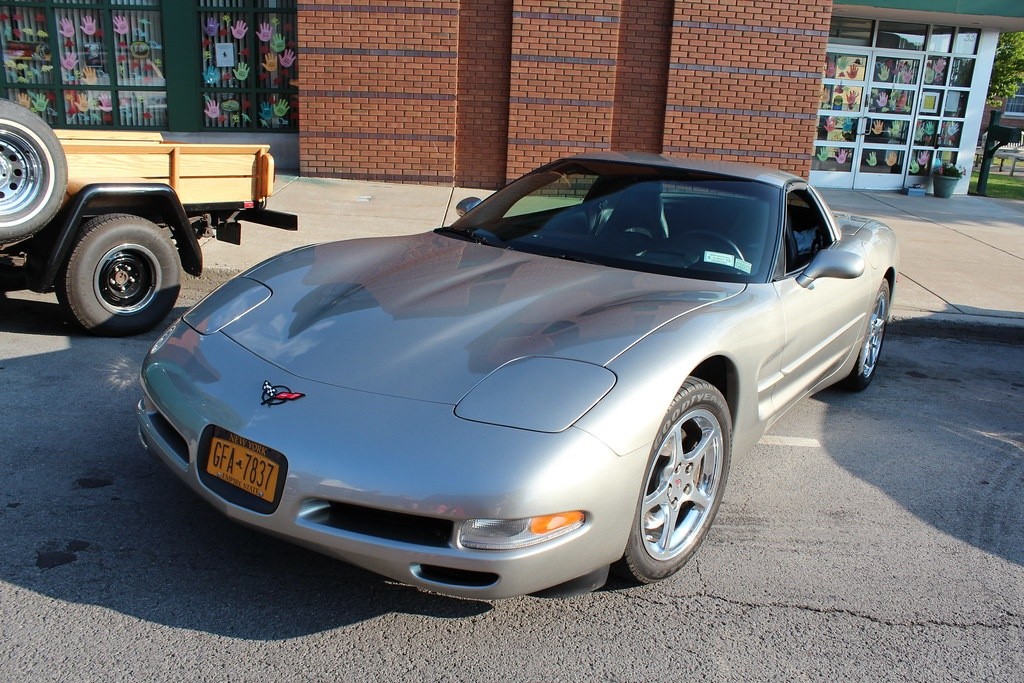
[975,149,1004,167]
[997,153,1024,177]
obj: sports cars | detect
[134,152,899,601]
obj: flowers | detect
[932,162,967,178]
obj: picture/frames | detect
[83,42,102,68]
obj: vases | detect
[933,174,962,199]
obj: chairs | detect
[511,172,670,262]
[638,196,786,279]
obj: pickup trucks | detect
[0,98,297,336]
